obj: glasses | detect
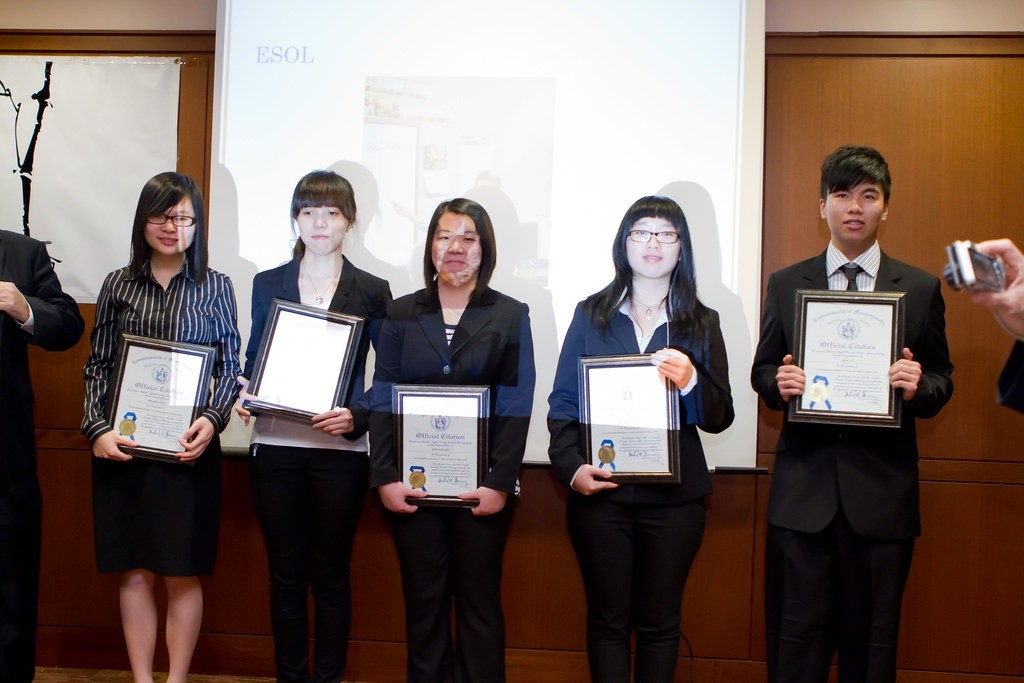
[145,212,197,227]
[626,229,682,244]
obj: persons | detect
[76,172,242,682]
[235,170,393,683]
[546,195,735,683]
[369,199,536,683]
[751,143,952,683]
[0,231,85,683]
[973,238,1024,412]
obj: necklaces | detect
[302,266,341,303]
[635,298,658,321]
[442,298,461,316]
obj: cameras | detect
[943,239,1004,292]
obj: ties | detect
[837,262,865,291]
